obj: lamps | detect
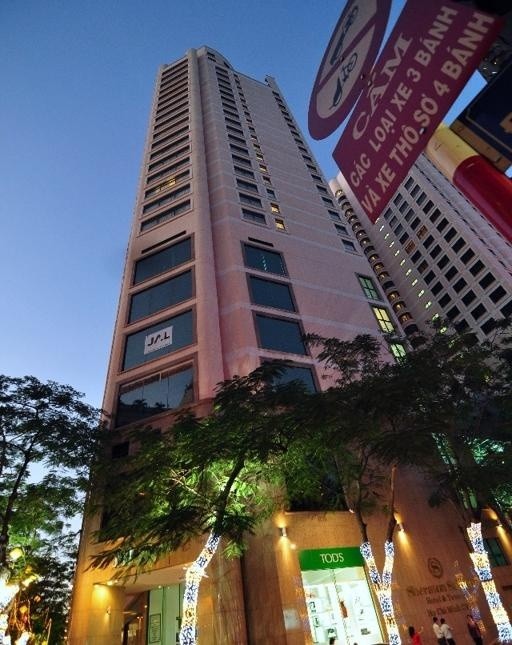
[495,519,503,526]
[396,522,406,532]
[278,526,290,538]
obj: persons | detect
[440,618,456,645]
[466,614,484,645]
[408,623,424,645]
[432,617,447,645]
